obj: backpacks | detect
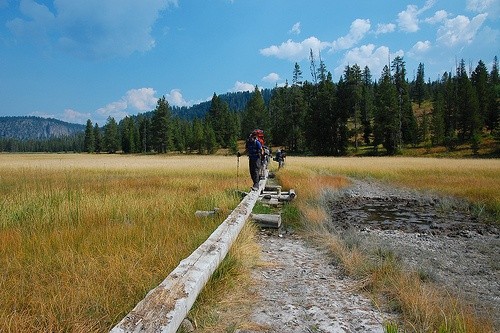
[254,129,265,158]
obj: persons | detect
[259,146,287,175]
[237,134,265,191]
